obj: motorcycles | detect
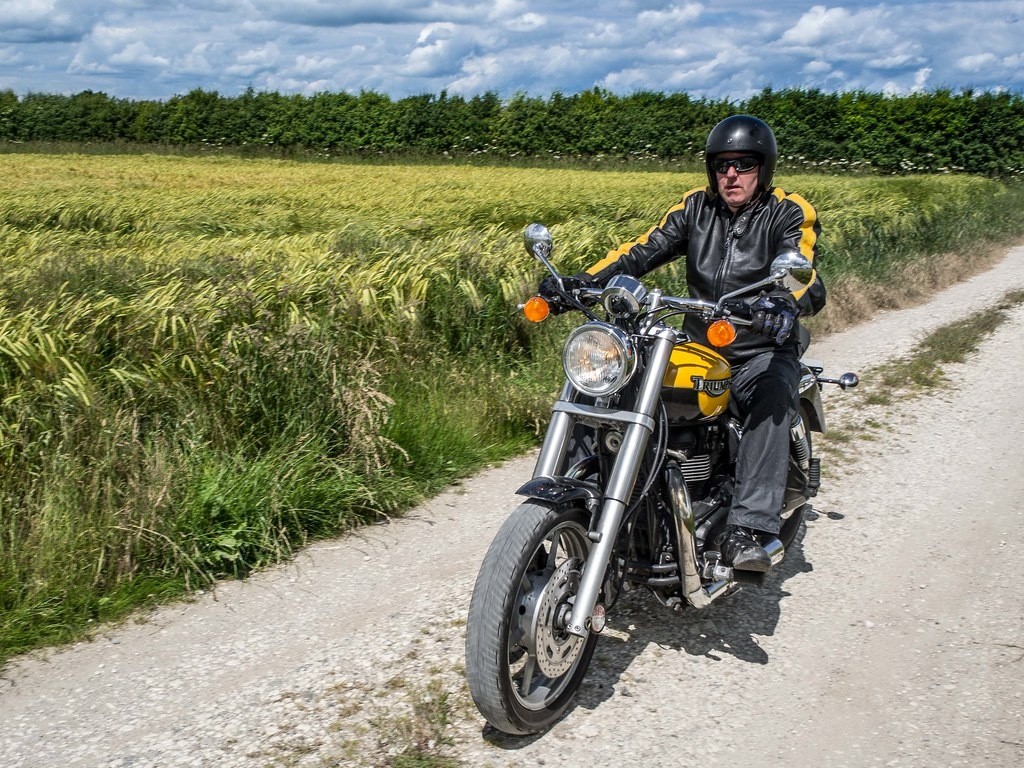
[461,219,863,739]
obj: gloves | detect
[751,286,802,345]
[538,272,602,316]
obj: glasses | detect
[710,155,761,173]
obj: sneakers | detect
[714,525,772,572]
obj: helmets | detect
[706,115,777,194]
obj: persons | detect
[536,115,821,574]
